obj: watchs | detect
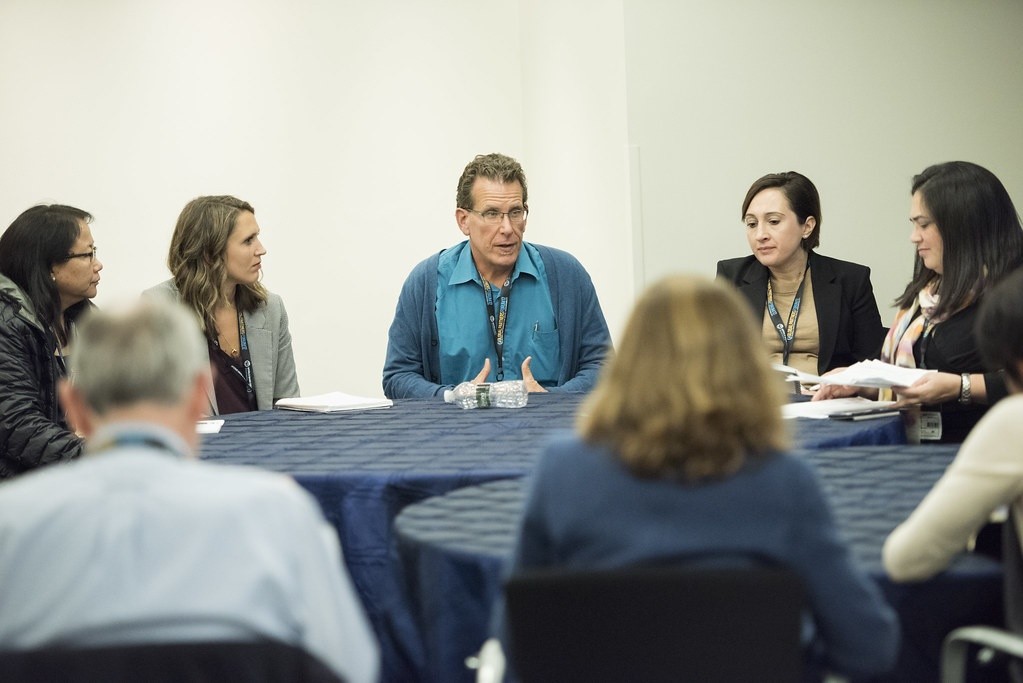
[958,372,972,407]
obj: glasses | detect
[466,208,529,222]
[52,247,97,262]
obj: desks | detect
[387,439,1023,683]
[190,390,910,652]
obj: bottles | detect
[443,381,528,409]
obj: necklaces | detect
[196,298,241,357]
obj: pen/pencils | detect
[809,383,820,391]
[534,320,539,332]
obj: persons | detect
[0,290,381,683]
[809,161,1023,446]
[382,153,616,401]
[882,269,1022,585]
[499,273,902,683]
[712,171,883,397]
[0,205,103,481]
[141,196,301,418]
